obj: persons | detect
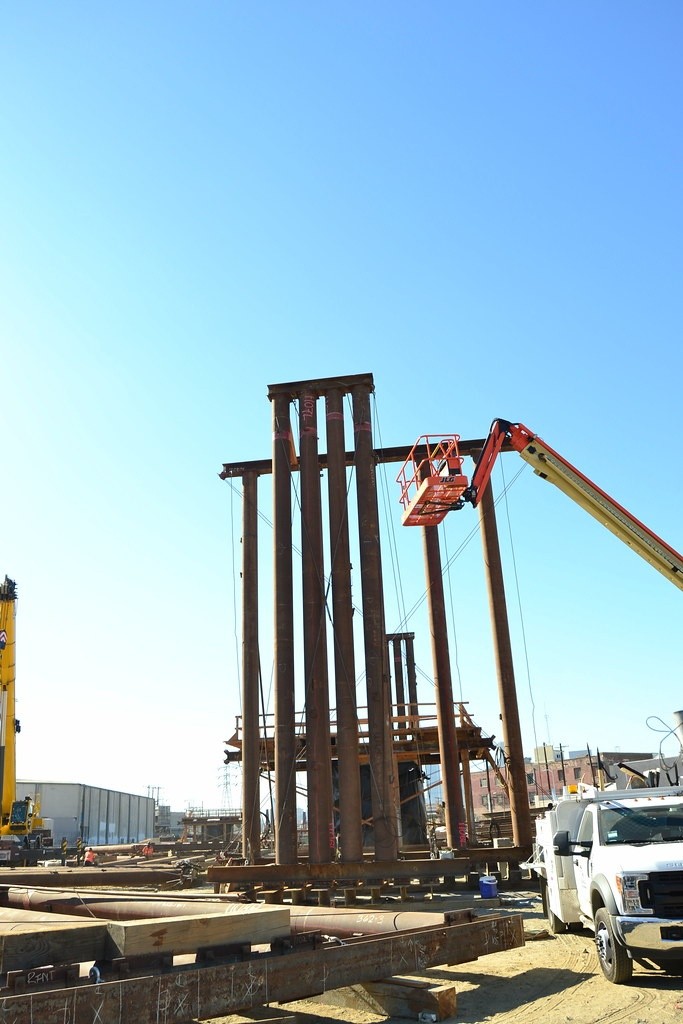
[83,847,94,866]
[140,842,154,860]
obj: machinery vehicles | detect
[397,414,683,597]
[0,575,86,868]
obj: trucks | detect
[518,780,683,984]
